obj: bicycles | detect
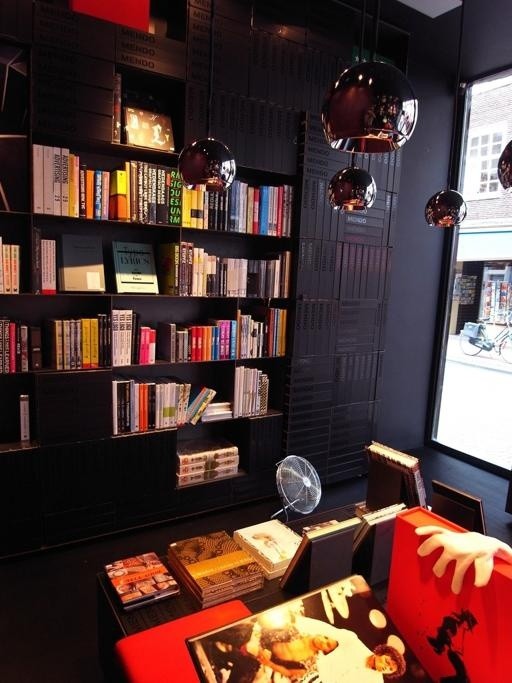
[459,312,512,363]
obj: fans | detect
[271,455,321,519]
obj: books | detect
[280,442,485,589]
[177,437,240,485]
[105,552,180,608]
[15,387,30,440]
[184,575,436,683]
[237,308,287,360]
[167,530,264,611]
[0,315,111,375]
[234,520,303,579]
[34,228,179,295]
[179,242,290,299]
[112,374,233,436]
[236,366,269,418]
[113,310,237,367]
[34,146,293,238]
[2,244,27,294]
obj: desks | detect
[100,484,511,683]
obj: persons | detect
[200,621,339,683]
[295,614,406,683]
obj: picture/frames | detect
[429,479,489,537]
